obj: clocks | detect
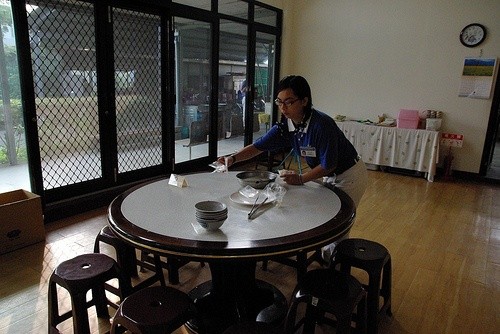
[460,23,486,47]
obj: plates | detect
[229,192,275,206]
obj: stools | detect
[255,136,284,170]
[48,225,393,334]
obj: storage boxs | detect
[0,188,46,254]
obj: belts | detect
[333,156,359,177]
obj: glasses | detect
[275,99,298,107]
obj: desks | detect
[106,171,358,334]
[336,121,440,181]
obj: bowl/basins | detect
[236,171,277,189]
[194,201,227,231]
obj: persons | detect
[217,75,368,272]
[223,91,227,103]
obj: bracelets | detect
[231,155,235,163]
[299,174,303,185]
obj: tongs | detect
[247,196,268,219]
